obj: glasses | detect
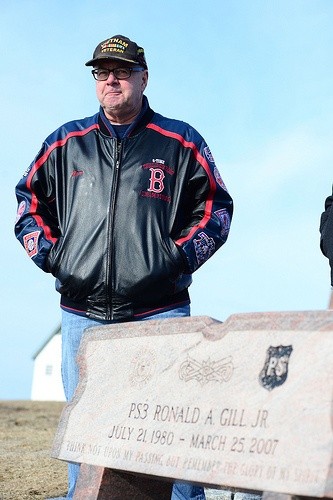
[91,68,143,81]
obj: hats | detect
[84,35,147,70]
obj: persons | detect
[320,184,333,290]
[14,35,233,500]
